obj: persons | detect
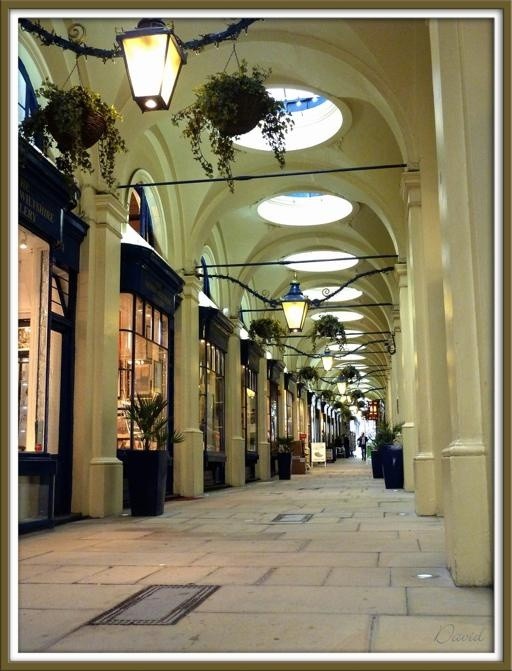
[342,434,349,458]
[335,436,342,447]
[357,432,369,461]
[322,430,325,442]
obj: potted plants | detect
[311,288,347,354]
[19,23,128,188]
[171,45,295,194]
[247,290,286,353]
[368,437,383,478]
[299,366,365,420]
[376,419,405,489]
[124,392,184,516]
[276,437,294,479]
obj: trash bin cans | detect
[381,444,404,489]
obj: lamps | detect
[280,272,311,332]
[321,345,336,371]
[116,18,187,112]
